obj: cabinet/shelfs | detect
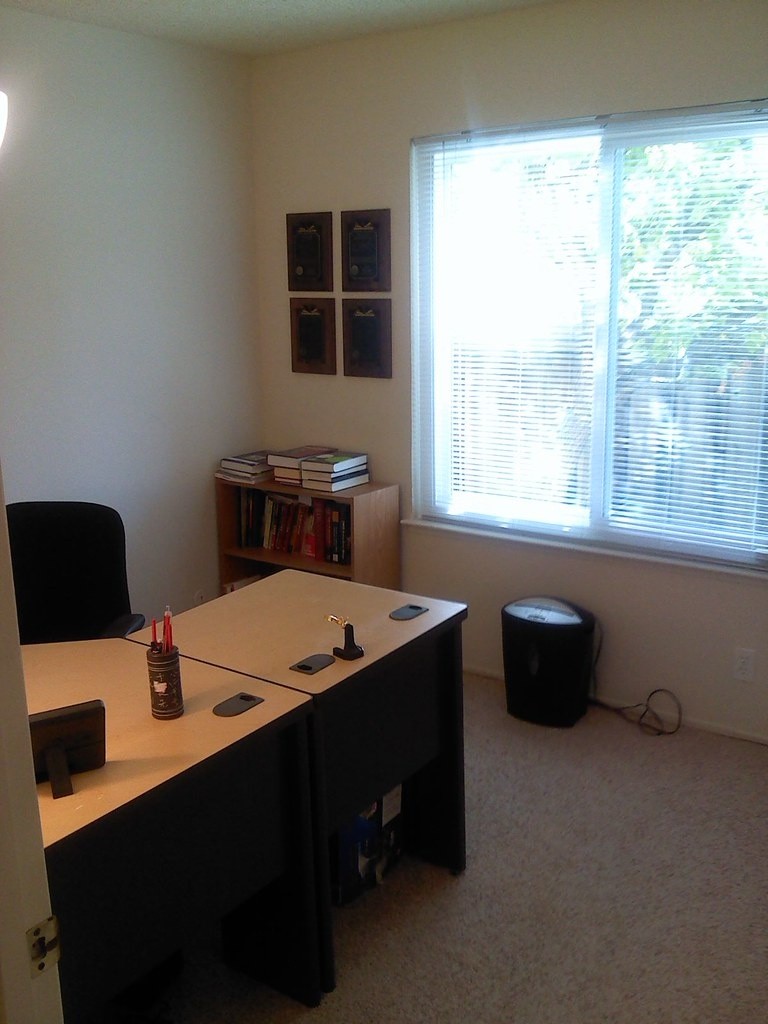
[215,477,399,596]
[19,569,468,1024]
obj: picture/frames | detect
[342,299,392,379]
[286,212,333,292]
[290,297,336,375]
[341,208,391,292]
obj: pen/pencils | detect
[168,617,173,651]
[162,603,173,655]
[151,618,158,652]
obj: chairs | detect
[6,501,145,645]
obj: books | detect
[212,443,371,595]
